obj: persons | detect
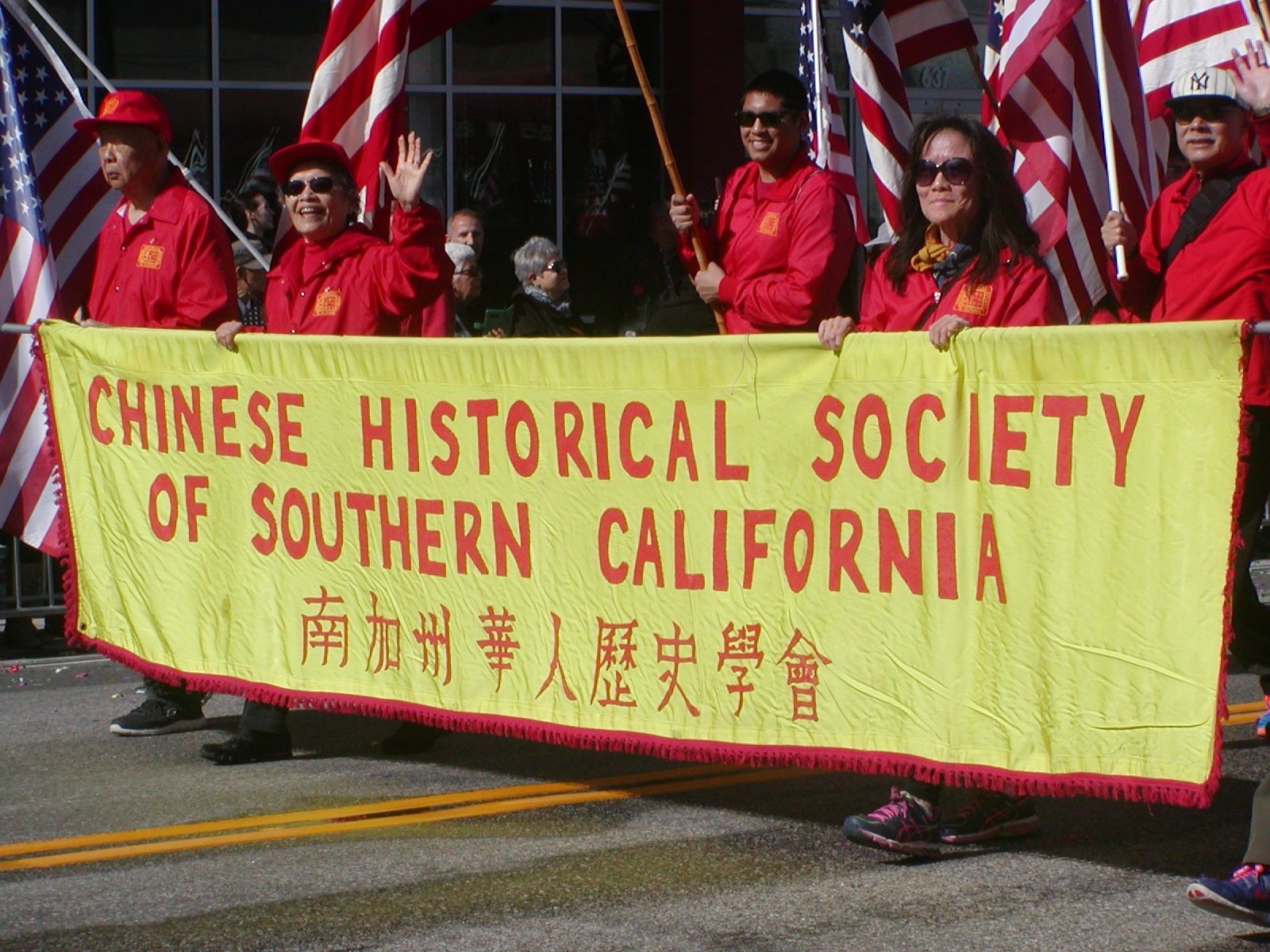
[818,119,1062,849]
[1099,38,1270,928]
[668,69,855,334]
[73,130,585,761]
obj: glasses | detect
[454,265,482,277]
[735,110,790,128]
[911,157,982,186]
[544,260,566,274]
[282,177,345,197]
[1171,102,1233,125]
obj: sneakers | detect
[939,792,1043,845]
[199,729,291,763]
[109,697,207,736]
[843,785,942,860]
[1186,865,1270,929]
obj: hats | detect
[232,240,273,269]
[1164,65,1243,109]
[268,139,355,185]
[72,90,171,145]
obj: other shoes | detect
[4,629,49,646]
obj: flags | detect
[798,0,1270,325]
[270,0,496,275]
[468,123,640,245]
[0,0,126,561]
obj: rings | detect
[1259,63,1267,66]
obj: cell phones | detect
[483,309,505,337]
